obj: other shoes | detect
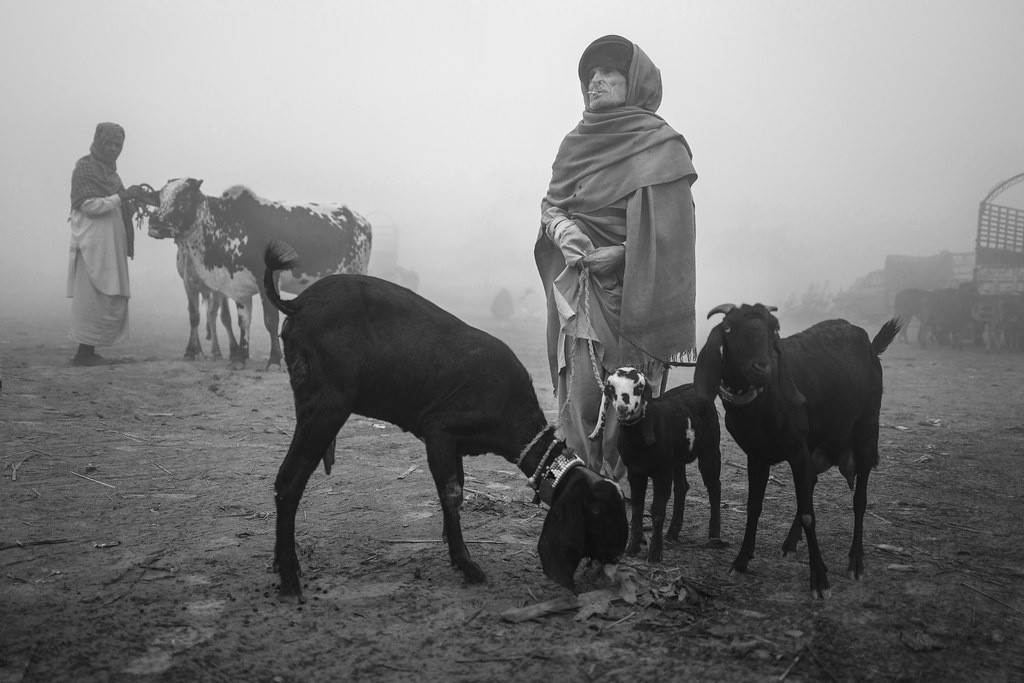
[74,352,113,366]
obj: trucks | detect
[970,173,1024,349]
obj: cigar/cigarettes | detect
[587,91,598,94]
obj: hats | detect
[578,35,634,88]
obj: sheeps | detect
[707,302,905,601]
[893,280,1023,353]
[260,243,632,605]
[602,366,724,562]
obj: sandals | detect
[625,503,653,530]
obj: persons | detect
[66,122,145,368]
[534,33,697,530]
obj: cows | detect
[149,176,370,369]
